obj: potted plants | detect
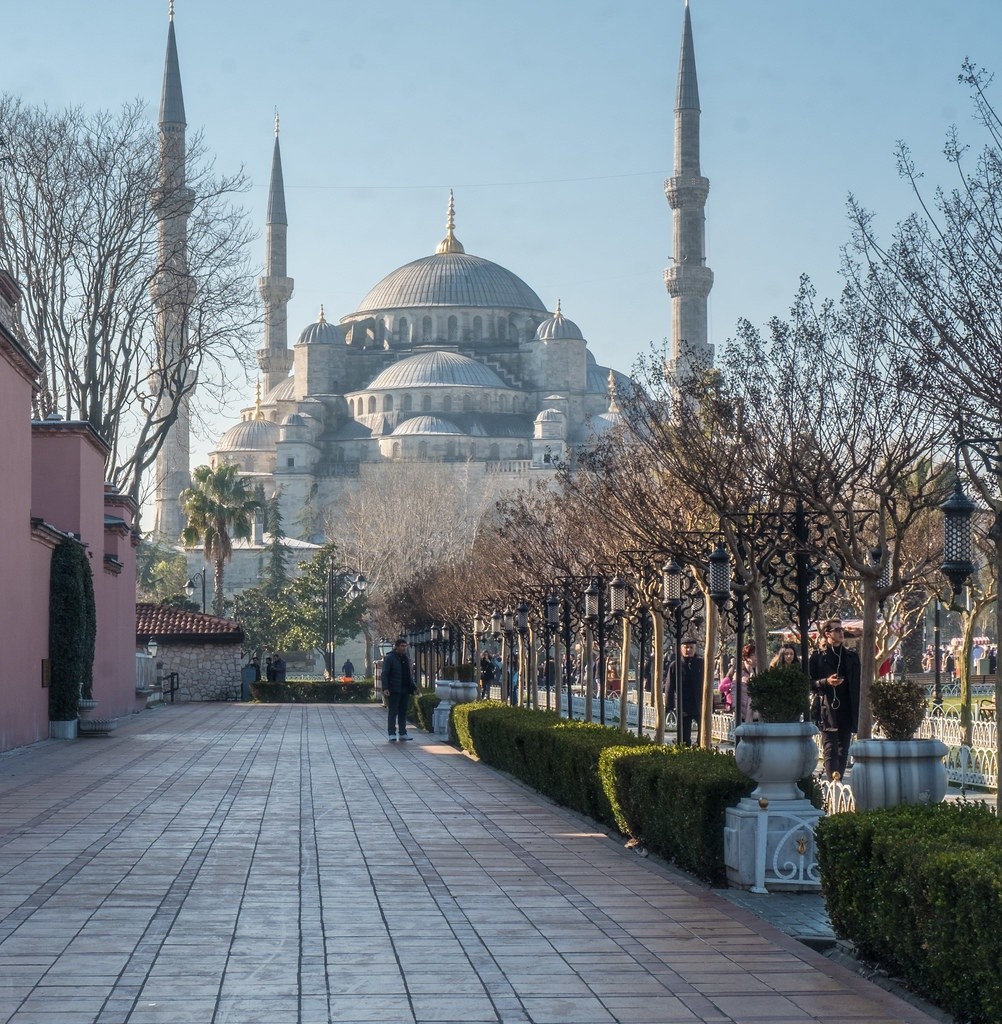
[435,664,456,706]
[49,539,101,740]
[451,663,479,703]
[851,676,950,813]
[737,666,819,800]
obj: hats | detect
[681,638,696,644]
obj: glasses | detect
[828,628,845,632]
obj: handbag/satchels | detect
[481,671,486,680]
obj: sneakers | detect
[389,734,397,741]
[399,734,413,740]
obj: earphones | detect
[828,635,831,637]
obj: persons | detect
[645,619,860,783]
[342,659,354,677]
[888,640,997,674]
[478,647,608,708]
[249,657,261,682]
[381,639,420,742]
[265,655,286,682]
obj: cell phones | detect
[836,676,845,680]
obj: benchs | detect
[970,675,997,685]
[907,672,950,689]
[713,694,735,715]
[979,691,997,722]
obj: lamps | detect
[148,637,158,658]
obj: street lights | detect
[326,565,370,682]
[378,438,1002,822]
[183,574,205,614]
